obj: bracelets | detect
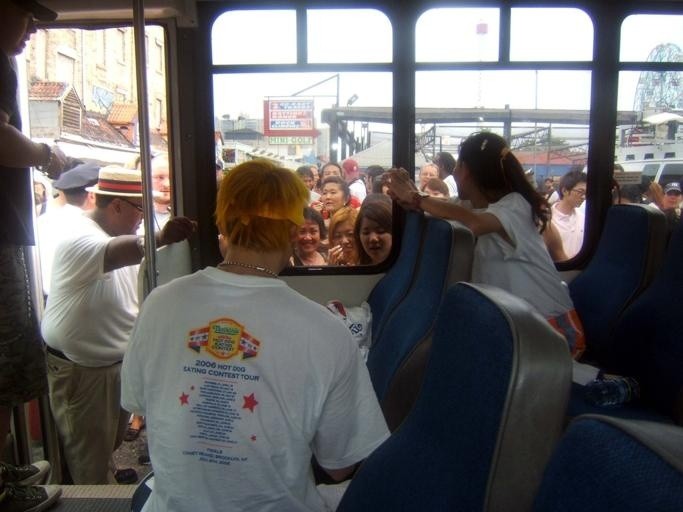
[37,142,53,176]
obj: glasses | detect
[569,188,586,197]
[119,196,144,213]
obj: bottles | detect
[583,374,643,410]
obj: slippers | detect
[124,428,139,441]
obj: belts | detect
[46,346,123,366]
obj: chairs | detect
[366,209,437,347]
[532,413,683,512]
[602,217,683,410]
[570,203,669,365]
[335,283,571,512]
[364,216,476,433]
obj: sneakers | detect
[0,461,49,484]
[0,483,62,512]
[115,468,138,484]
[139,455,151,466]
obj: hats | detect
[664,182,682,194]
[11,1,58,21]
[343,160,359,179]
[524,168,534,176]
[54,163,99,195]
[85,167,162,198]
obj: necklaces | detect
[214,261,280,281]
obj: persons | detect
[134,154,173,241]
[33,161,104,312]
[338,160,360,187]
[655,182,683,216]
[316,177,352,228]
[372,172,392,193]
[363,163,384,193]
[0,0,68,489]
[328,207,360,268]
[420,178,451,218]
[318,162,342,185]
[415,163,440,196]
[545,172,587,258]
[295,166,322,205]
[612,181,659,210]
[353,198,390,265]
[378,132,585,359]
[120,157,391,512]
[429,151,456,179]
[42,164,145,485]
[295,206,334,268]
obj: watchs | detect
[407,190,432,207]
